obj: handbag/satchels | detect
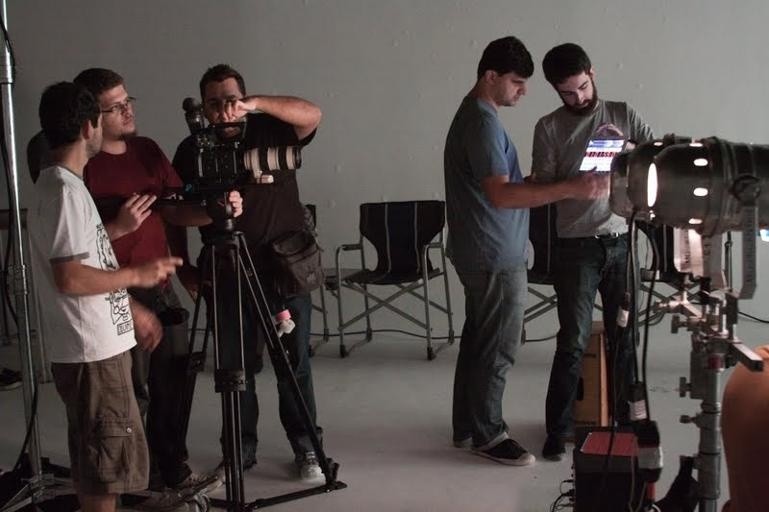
[273,227,328,292]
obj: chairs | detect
[521,203,557,346]
[334,200,453,360]
[304,203,360,358]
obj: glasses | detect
[101,96,136,114]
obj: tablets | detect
[576,136,630,174]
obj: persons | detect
[443,38,609,464]
[530,42,654,463]
[170,66,320,482]
[27,68,242,511]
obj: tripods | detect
[179,230,338,509]
[0,31,160,512]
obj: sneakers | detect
[542,435,568,461]
[168,470,224,491]
[212,450,259,485]
[453,432,536,466]
[297,449,325,481]
